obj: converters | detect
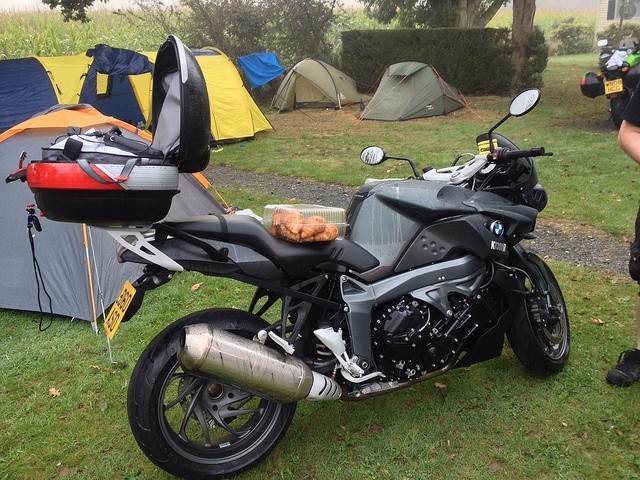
[67,125,82,135]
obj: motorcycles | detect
[580,40,640,129]
[26,35,571,480]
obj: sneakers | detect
[607,345,640,387]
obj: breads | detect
[269,208,337,241]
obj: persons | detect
[606,76,640,385]
